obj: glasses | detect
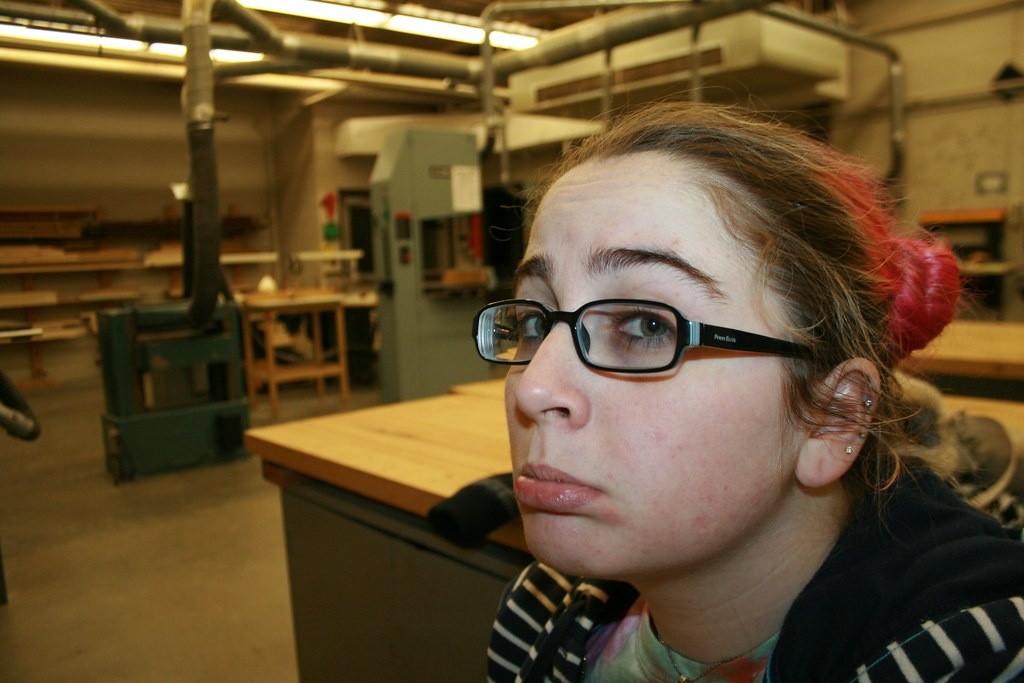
[472,299,840,373]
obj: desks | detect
[241,288,353,419]
[901,315,1024,399]
[238,375,1024,683]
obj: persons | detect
[469,100,1024,683]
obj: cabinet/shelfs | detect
[0,263,143,373]
[147,243,363,299]
